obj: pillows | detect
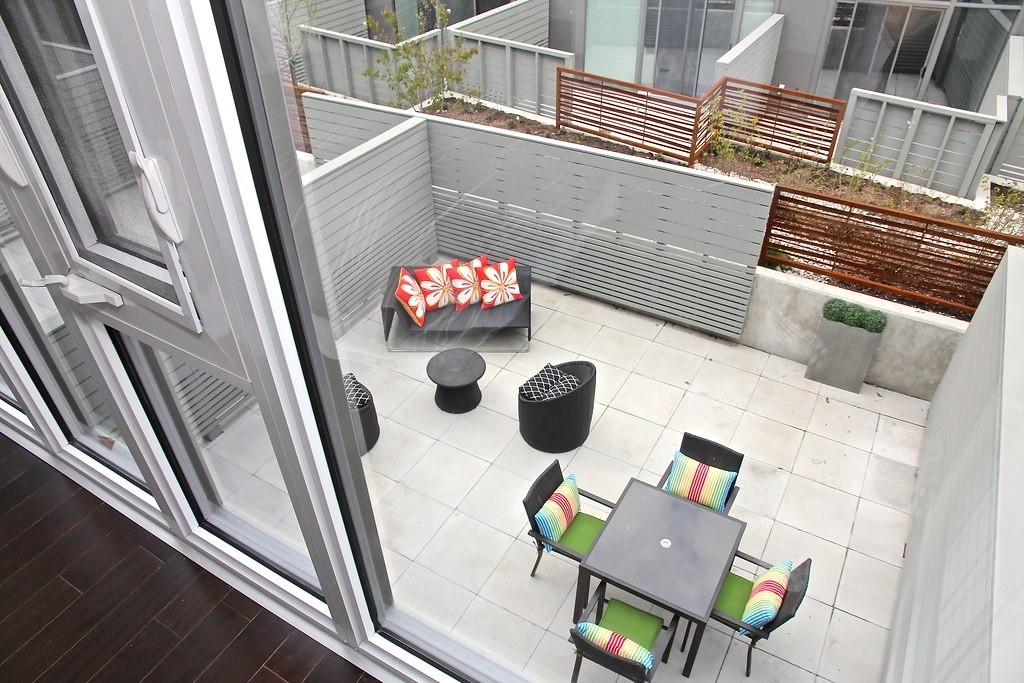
[395,267,426,327]
[414,259,459,310]
[475,256,523,310]
[739,560,793,636]
[576,622,654,671]
[534,472,581,552]
[519,363,581,402]
[342,373,370,412]
[663,450,738,514]
[446,253,488,311]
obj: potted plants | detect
[804,299,887,394]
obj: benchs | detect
[380,266,531,342]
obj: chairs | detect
[519,361,595,454]
[680,548,811,676]
[342,372,380,456]
[568,581,680,682]
[522,459,616,577]
[657,432,744,516]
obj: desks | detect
[573,477,748,677]
[426,347,486,413]
